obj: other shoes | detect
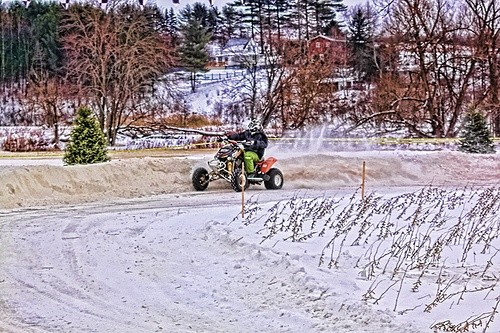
[247,173,255,178]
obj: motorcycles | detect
[192,136,284,190]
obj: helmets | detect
[248,120,263,135]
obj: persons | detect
[218,120,268,177]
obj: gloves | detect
[220,136,228,140]
[246,140,254,146]
